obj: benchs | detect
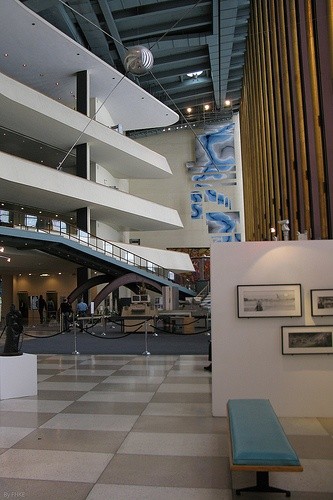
[224,399,304,500]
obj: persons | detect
[20,299,26,318]
[39,295,47,324]
[204,334,212,372]
[48,296,55,320]
[60,297,72,332]
[76,299,88,332]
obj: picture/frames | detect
[281,325,333,355]
[236,283,303,319]
[310,288,333,317]
